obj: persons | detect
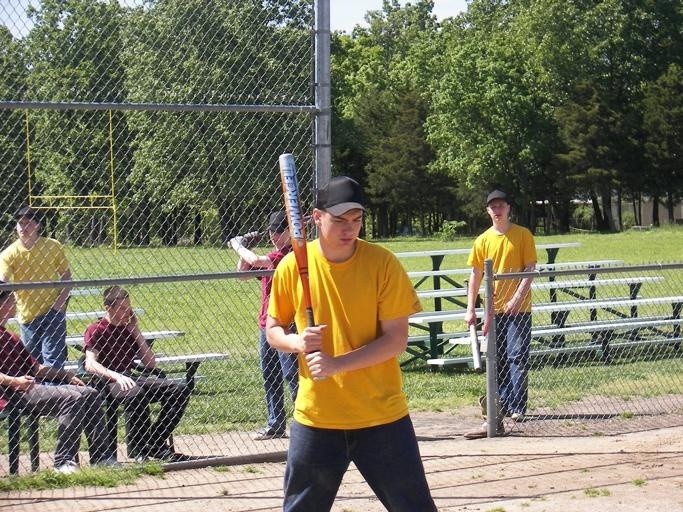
[265,176,437,511]
[82,287,191,462]
[0,206,73,386]
[466,189,538,438]
[231,210,298,441]
[1,280,122,474]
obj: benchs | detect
[392,240,683,373]
[0,288,229,475]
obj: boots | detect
[464,395,505,439]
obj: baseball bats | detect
[226,216,314,248]
[278,154,326,381]
[464,278,483,372]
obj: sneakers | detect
[52,462,83,474]
[253,429,286,440]
[127,450,190,464]
[511,412,524,420]
[91,458,124,470]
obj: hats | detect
[267,211,289,232]
[315,176,368,217]
[15,207,37,219]
[486,190,509,205]
